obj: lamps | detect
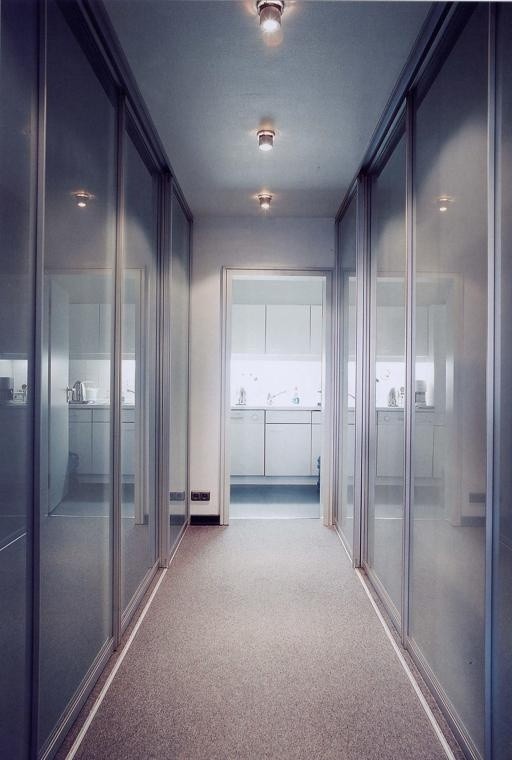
[258,193,272,209]
[438,195,449,211]
[257,129,275,152]
[256,0,284,32]
[73,192,91,207]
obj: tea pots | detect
[74,380,87,402]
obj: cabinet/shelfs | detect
[434,410,447,487]
[69,404,135,484]
[230,407,356,485]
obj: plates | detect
[69,400,95,405]
